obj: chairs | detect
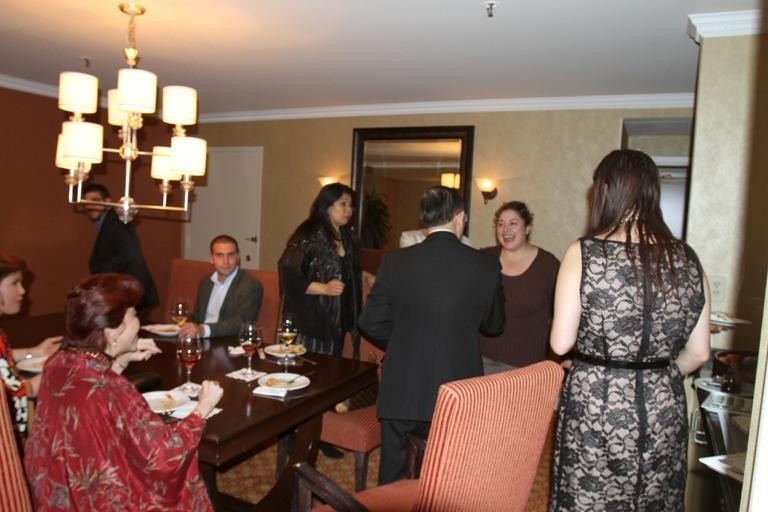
[293,335,390,489]
[290,360,565,510]
[0,378,32,511]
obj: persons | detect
[357,185,507,492]
[0,251,68,442]
[476,197,558,377]
[278,181,359,459]
[398,213,476,253]
[548,148,713,511]
[81,184,162,319]
[177,231,263,342]
[19,273,223,512]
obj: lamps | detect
[439,172,461,189]
[317,174,338,189]
[44,3,209,223]
[477,173,497,200]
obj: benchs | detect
[166,258,286,342]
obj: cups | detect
[170,300,191,327]
[722,374,741,394]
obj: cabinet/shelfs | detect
[688,343,759,512]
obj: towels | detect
[251,386,287,398]
[169,400,223,421]
[224,367,266,383]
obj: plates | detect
[708,313,752,329]
[730,412,751,432]
[254,372,312,393]
[699,449,744,482]
[699,390,751,417]
[714,350,757,371]
[262,342,308,359]
[140,322,178,337]
[13,357,50,375]
[694,375,753,398]
[140,390,192,415]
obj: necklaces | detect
[611,214,647,226]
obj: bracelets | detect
[25,346,33,360]
[113,359,126,370]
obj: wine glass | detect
[274,314,299,367]
[237,321,264,377]
[174,331,203,395]
